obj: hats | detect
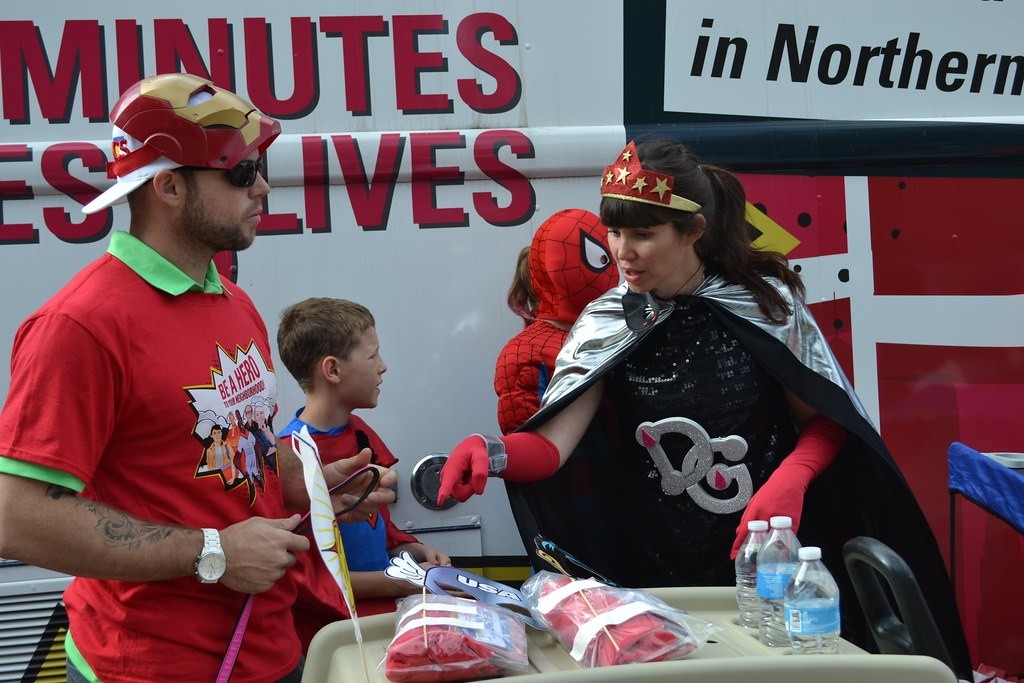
[79,81,240,215]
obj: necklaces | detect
[643,262,702,326]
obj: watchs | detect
[194,527,228,583]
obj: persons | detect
[507,245,540,328]
[439,133,973,683]
[494,209,621,434]
[275,297,474,659]
[0,73,397,683]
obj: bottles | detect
[735,520,768,628]
[782,547,842,655]
[756,516,804,647]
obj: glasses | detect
[175,156,269,189]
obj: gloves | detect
[434,431,561,506]
[729,416,852,563]
